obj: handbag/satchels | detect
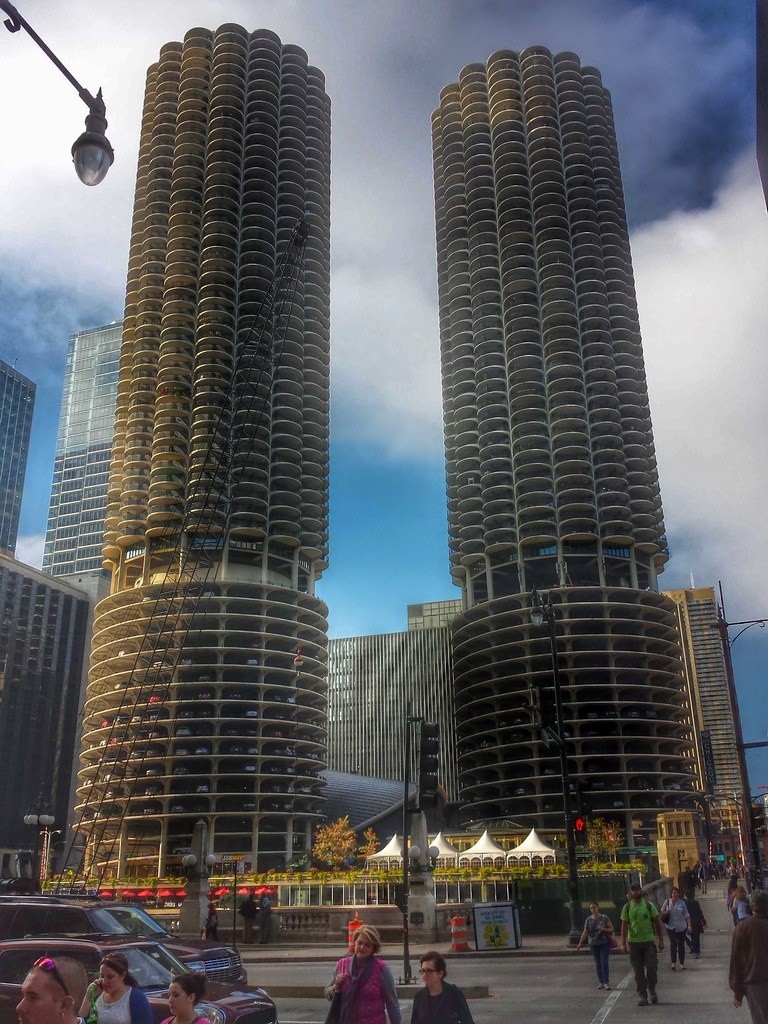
[661,898,670,925]
[745,896,753,915]
[609,934,618,948]
[201,927,206,941]
[324,958,346,1024]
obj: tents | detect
[367,834,404,870]
[507,828,557,867]
[458,829,506,867]
[429,832,458,867]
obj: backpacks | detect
[627,889,651,914]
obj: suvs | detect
[0,932,278,1024]
[0,894,249,986]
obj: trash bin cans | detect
[473,901,526,950]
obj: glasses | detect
[419,968,442,974]
[33,957,69,997]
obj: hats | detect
[630,882,641,890]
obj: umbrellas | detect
[98,890,186,903]
[214,887,272,896]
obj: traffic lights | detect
[575,788,591,833]
[416,720,441,810]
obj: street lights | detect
[23,783,56,891]
[528,585,588,948]
[40,829,61,880]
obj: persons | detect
[576,902,614,989]
[729,889,768,1024]
[661,887,692,971]
[16,957,87,1024]
[686,861,754,894]
[726,880,737,926]
[323,925,403,1024]
[679,889,705,959]
[78,953,153,1024]
[205,903,219,942]
[243,894,256,943]
[256,895,272,944]
[411,952,475,1024]
[731,886,752,922]
[619,883,664,1005]
[161,973,212,1024]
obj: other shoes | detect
[689,942,693,954]
[681,964,686,970]
[671,966,676,971]
[694,953,698,959]
[604,983,611,990]
[597,982,604,989]
[649,991,658,1003]
[637,999,648,1006]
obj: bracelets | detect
[621,944,625,945]
